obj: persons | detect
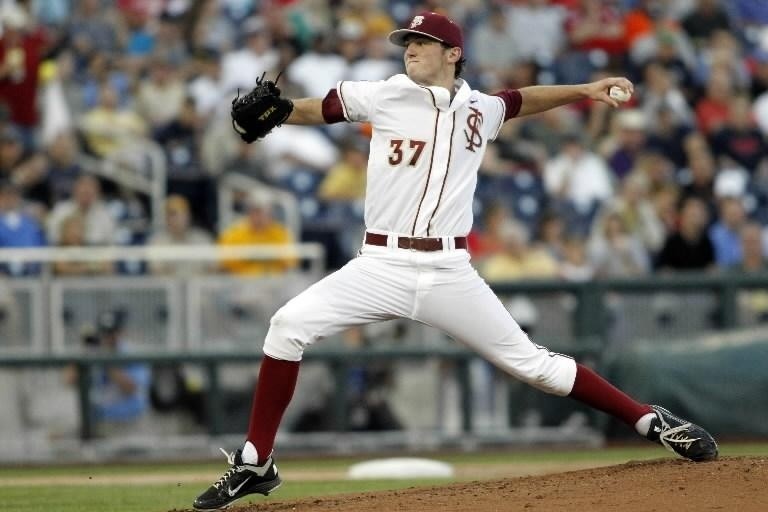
[192,9,720,511]
[0,1,768,444]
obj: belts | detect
[364,231,470,251]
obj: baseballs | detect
[609,86,632,104]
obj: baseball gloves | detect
[231,72,294,145]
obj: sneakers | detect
[193,440,284,509]
[645,401,718,463]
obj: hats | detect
[388,11,464,53]
